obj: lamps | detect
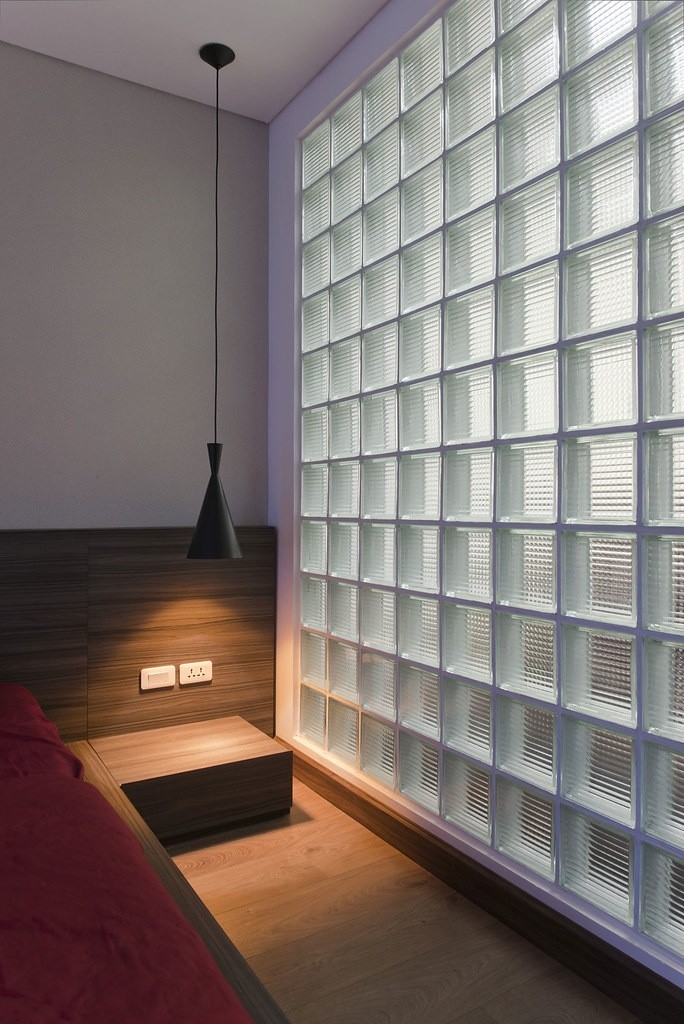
[182,43,245,559]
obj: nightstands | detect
[87,720,293,839]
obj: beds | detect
[1,681,295,1024]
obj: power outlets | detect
[179,661,212,684]
